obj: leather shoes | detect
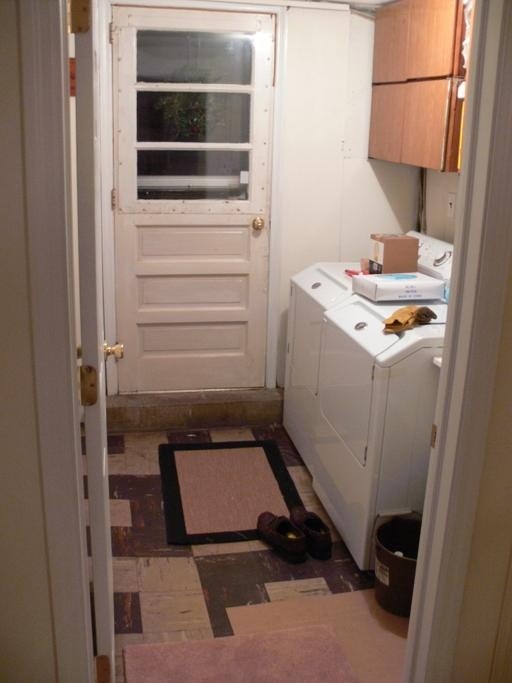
[258,511,307,564]
[290,506,331,561]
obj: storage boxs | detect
[368,231,420,275]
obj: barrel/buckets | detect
[370,508,422,620]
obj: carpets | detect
[158,439,306,545]
[123,628,359,681]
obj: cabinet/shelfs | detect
[366,0,465,173]
